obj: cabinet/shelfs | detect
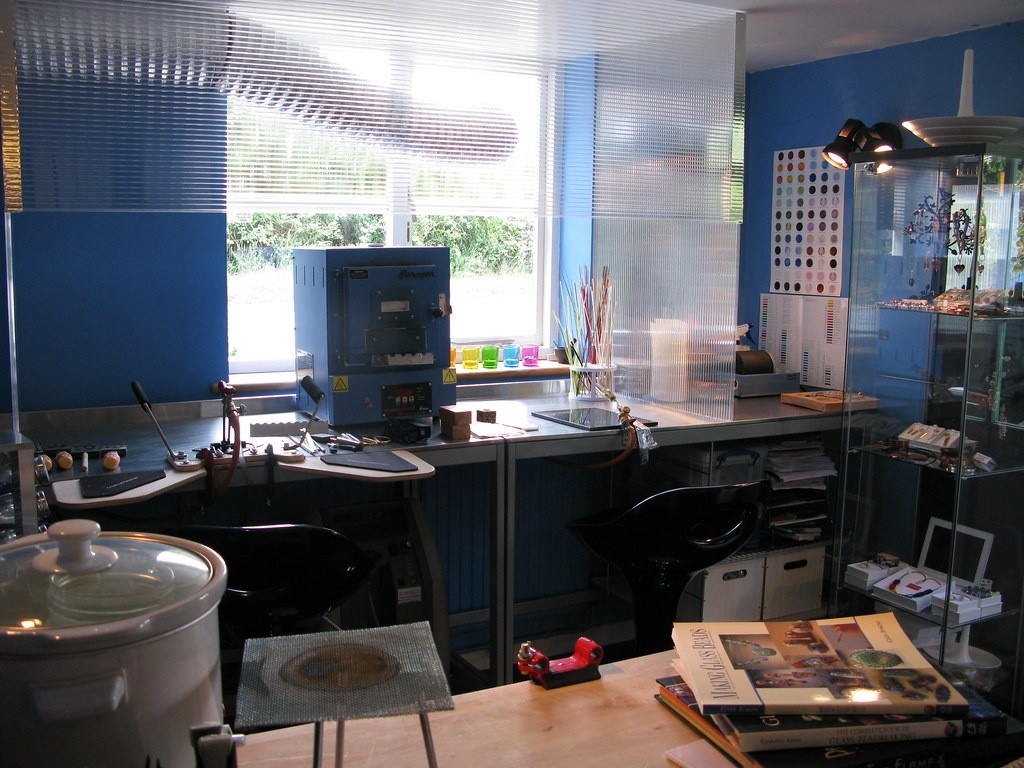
[824,141,1024,716]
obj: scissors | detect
[360,428,391,445]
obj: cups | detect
[522,344,539,365]
[450,347,457,368]
[503,345,520,367]
[463,346,479,369]
[482,346,499,369]
[554,347,574,364]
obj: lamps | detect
[820,119,904,176]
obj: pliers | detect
[327,442,357,453]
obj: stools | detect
[567,477,770,657]
[164,524,384,646]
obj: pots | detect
[0,518,227,768]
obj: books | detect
[652,611,1007,768]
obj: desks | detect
[232,646,745,768]
